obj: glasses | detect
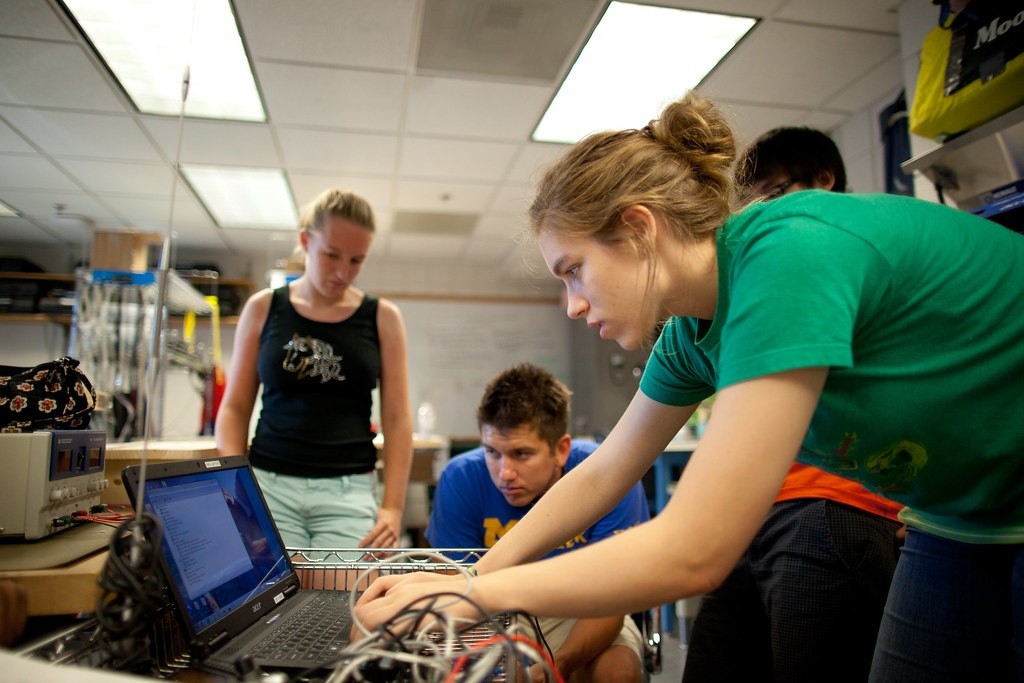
[765,178,797,199]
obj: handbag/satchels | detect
[0,355,97,432]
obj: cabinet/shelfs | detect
[1,269,260,325]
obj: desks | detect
[103,437,452,510]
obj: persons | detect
[681,126,908,683]
[350,93,1024,683]
[215,189,413,562]
[426,363,653,683]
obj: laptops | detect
[123,455,410,681]
